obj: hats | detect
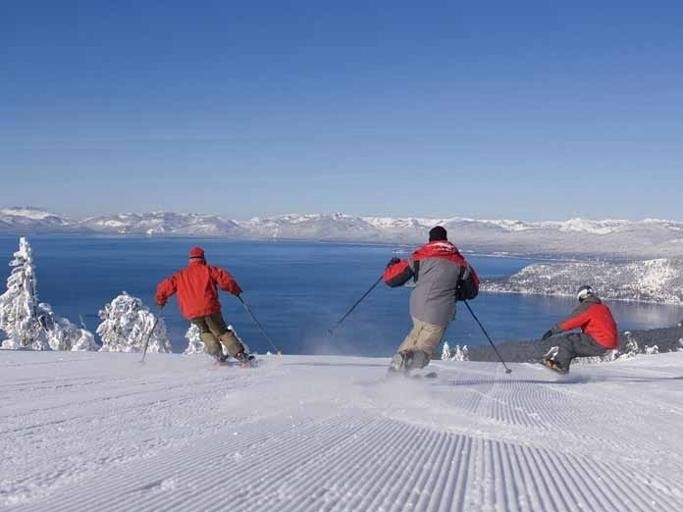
[189,247,204,257]
[429,226,447,240]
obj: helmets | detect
[576,285,594,301]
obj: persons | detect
[538,284,618,376]
[154,247,255,363]
[383,227,479,374]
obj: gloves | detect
[390,257,400,264]
[542,331,552,341]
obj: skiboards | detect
[209,355,257,369]
[352,370,439,388]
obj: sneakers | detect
[218,354,229,363]
[388,349,414,372]
[235,351,255,367]
[545,359,570,373]
